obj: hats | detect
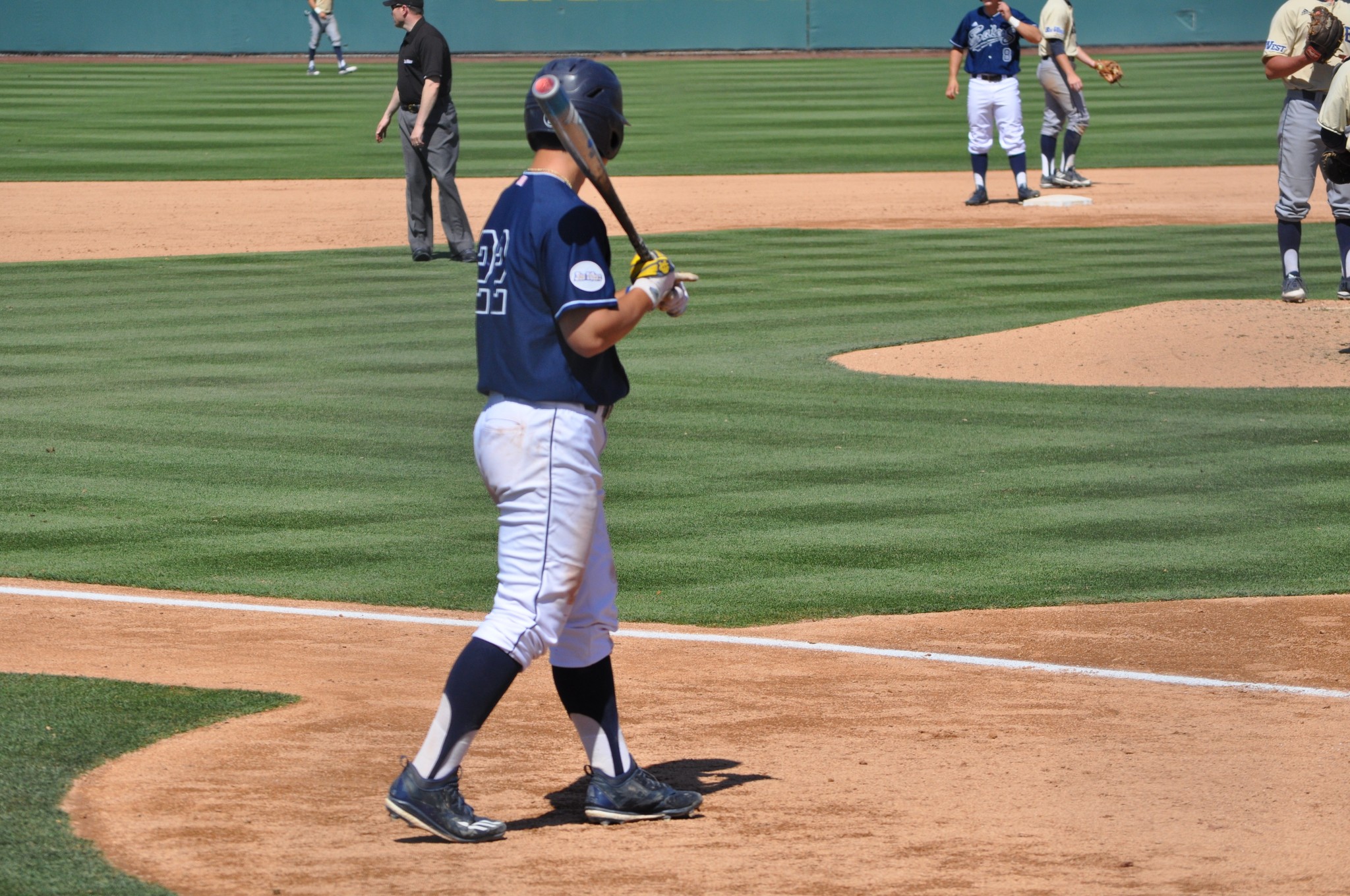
[383,0,424,8]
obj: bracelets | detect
[314,7,321,14]
[1008,16,1020,28]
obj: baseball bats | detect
[531,73,687,317]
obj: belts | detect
[1042,55,1074,61]
[584,401,612,419]
[401,104,418,113]
[971,73,1012,82]
[1302,89,1327,103]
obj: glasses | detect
[391,4,410,10]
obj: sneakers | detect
[1018,184,1039,202]
[1337,275,1350,300]
[452,248,478,262]
[384,761,507,843]
[583,752,703,825]
[1059,166,1091,187]
[1040,168,1080,188]
[412,249,431,261]
[1282,273,1306,303]
[965,188,989,206]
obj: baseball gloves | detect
[1304,6,1343,62]
[1096,60,1123,83]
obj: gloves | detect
[627,248,676,309]
[655,269,698,318]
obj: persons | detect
[374,0,479,263]
[1262,1,1350,349]
[383,54,702,843]
[945,0,1042,206]
[304,0,358,76]
[1035,0,1123,188]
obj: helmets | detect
[523,58,630,160]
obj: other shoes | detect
[306,69,320,75]
[339,66,357,74]
